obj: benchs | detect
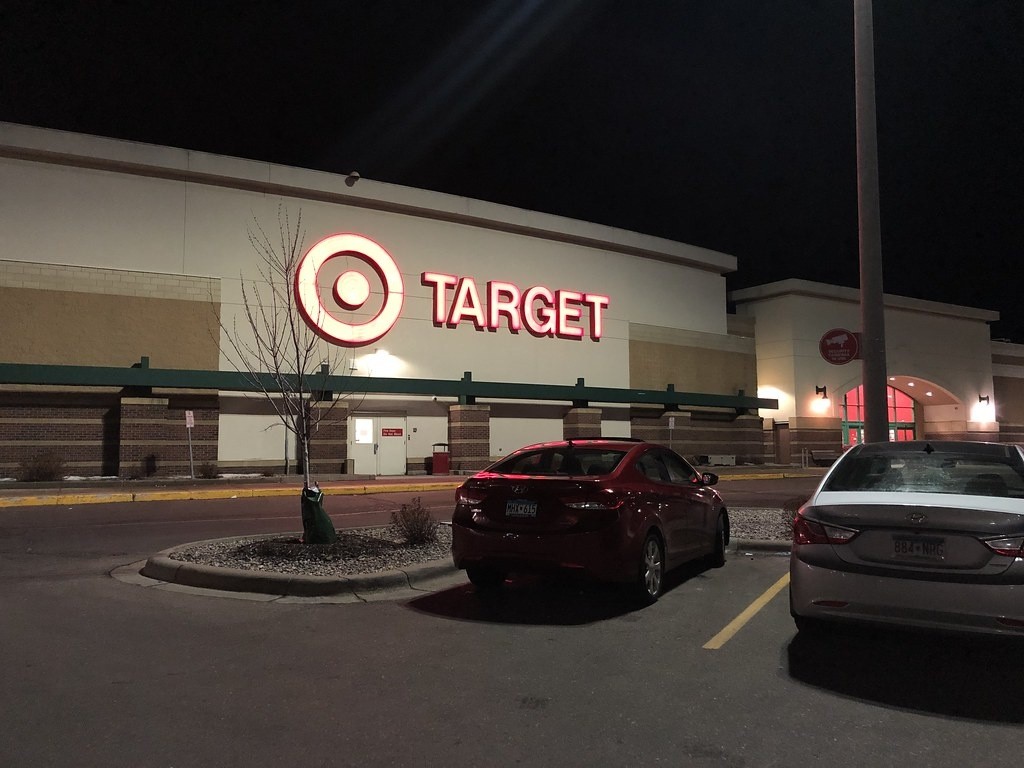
[811,450,842,467]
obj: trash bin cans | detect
[431,442,452,477]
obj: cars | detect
[786,437,1024,641]
[451,437,730,609]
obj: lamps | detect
[815,384,831,410]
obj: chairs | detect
[560,455,585,476]
[964,474,1008,496]
[861,467,904,491]
[587,464,609,477]
